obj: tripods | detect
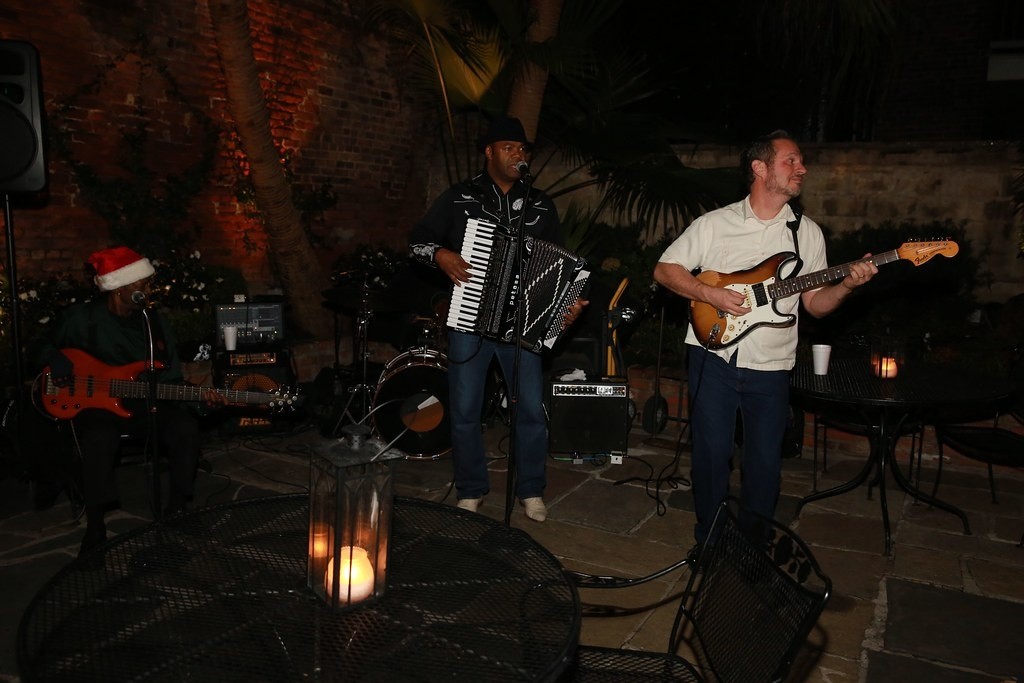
[333,275,373,438]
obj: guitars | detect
[687,234,960,351]
[39,345,306,423]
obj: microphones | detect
[131,290,146,310]
[516,161,531,177]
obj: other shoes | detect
[689,546,703,572]
[157,508,208,534]
[77,528,105,570]
[521,498,548,522]
[456,494,484,512]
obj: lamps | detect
[304,423,405,612]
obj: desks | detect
[787,358,972,555]
[18,494,579,683]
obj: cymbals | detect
[320,287,402,316]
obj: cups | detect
[812,344,831,375]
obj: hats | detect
[478,117,528,152]
[89,247,155,290]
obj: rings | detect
[858,274,865,279]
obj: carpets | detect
[858,645,1013,683]
[863,574,1024,683]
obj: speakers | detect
[0,39,51,209]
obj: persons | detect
[31,248,229,570]
[653,129,877,601]
[407,115,592,522]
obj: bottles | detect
[330,375,344,405]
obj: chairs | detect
[525,496,834,683]
[928,294,1024,550]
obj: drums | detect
[372,347,454,459]
